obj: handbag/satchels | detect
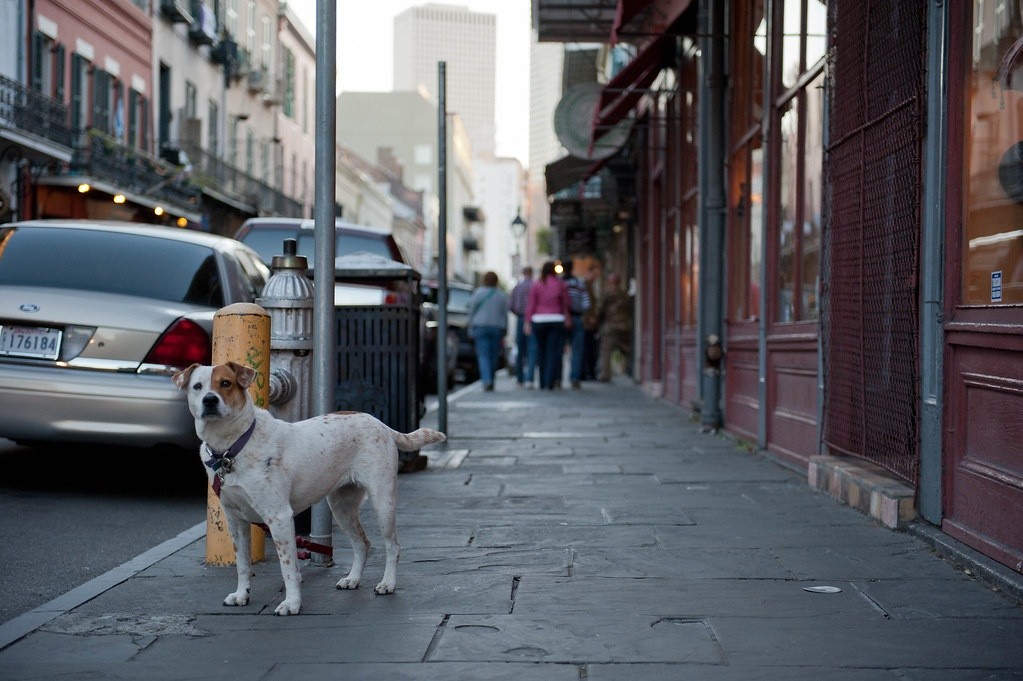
[465,324,474,339]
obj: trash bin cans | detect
[307,249,429,474]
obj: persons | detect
[464,271,509,392]
[509,258,636,392]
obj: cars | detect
[0,219,272,476]
[419,279,513,388]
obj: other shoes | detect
[553,383,560,390]
[547,384,554,390]
[570,382,580,390]
[483,383,494,393]
[595,378,610,382]
[525,382,534,389]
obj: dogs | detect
[172,361,447,617]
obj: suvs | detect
[233,214,438,398]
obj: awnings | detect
[35,173,204,226]
[610,0,668,48]
[544,152,611,198]
[587,33,673,159]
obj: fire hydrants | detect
[249,239,315,537]
